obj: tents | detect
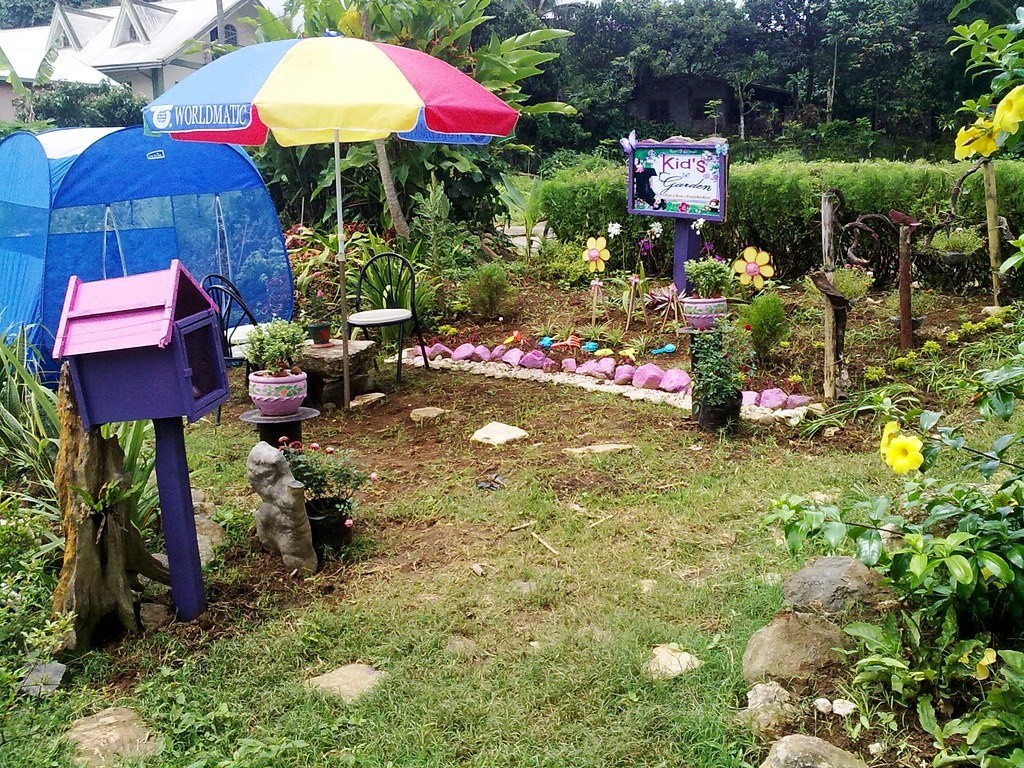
[0,126,294,385]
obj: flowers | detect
[734,246,774,289]
[606,221,622,239]
[279,436,377,527]
[799,263,876,304]
[303,272,328,324]
[582,237,611,274]
[701,242,725,262]
[692,324,752,405]
[628,274,638,285]
[638,238,653,256]
[646,221,663,240]
[691,217,707,236]
[590,278,603,292]
[918,227,986,259]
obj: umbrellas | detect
[139,26,521,416]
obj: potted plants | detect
[233,318,307,415]
[886,289,935,331]
[678,257,732,328]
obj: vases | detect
[698,387,743,433]
[936,249,965,263]
[304,497,353,554]
[308,323,332,344]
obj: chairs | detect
[346,252,431,386]
[200,273,264,426]
